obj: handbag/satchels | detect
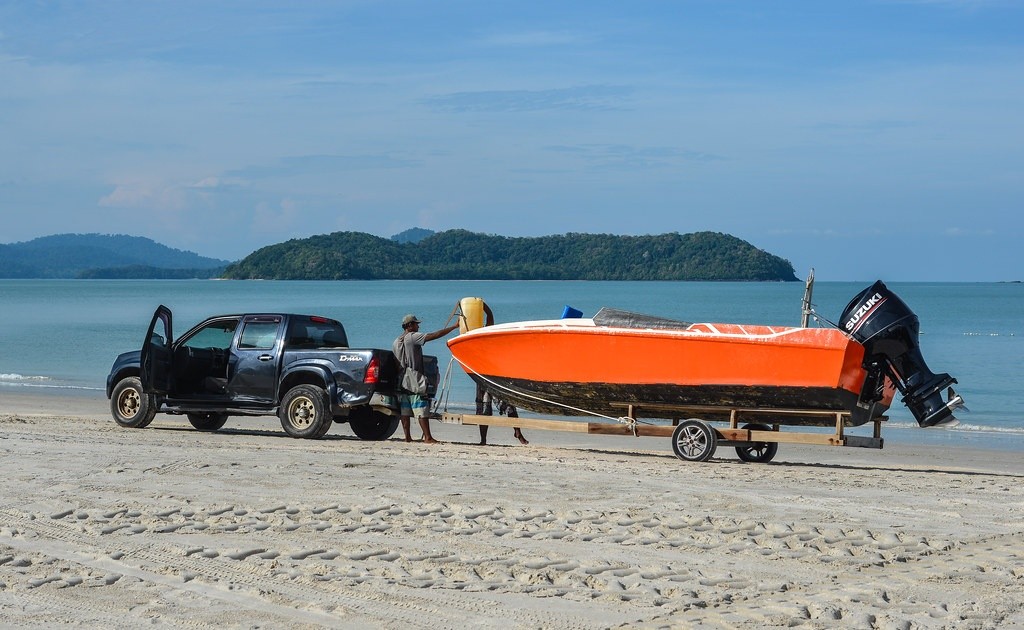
[400,366,430,397]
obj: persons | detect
[475,301,529,445]
[391,314,460,444]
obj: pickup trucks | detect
[105,304,442,439]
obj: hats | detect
[401,314,421,327]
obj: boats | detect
[445,279,970,428]
